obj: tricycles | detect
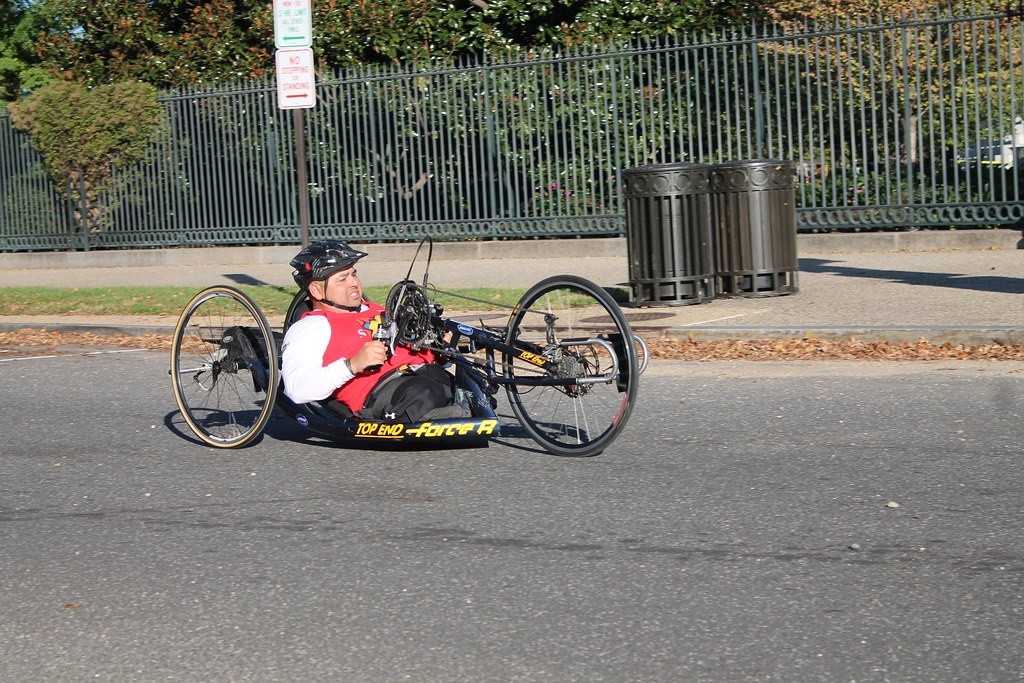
[167,234,649,450]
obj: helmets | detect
[289,238,369,291]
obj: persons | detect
[281,239,498,419]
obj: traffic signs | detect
[272,0,313,49]
[274,48,317,110]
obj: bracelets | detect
[344,358,355,376]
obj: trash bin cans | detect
[620,159,798,309]
